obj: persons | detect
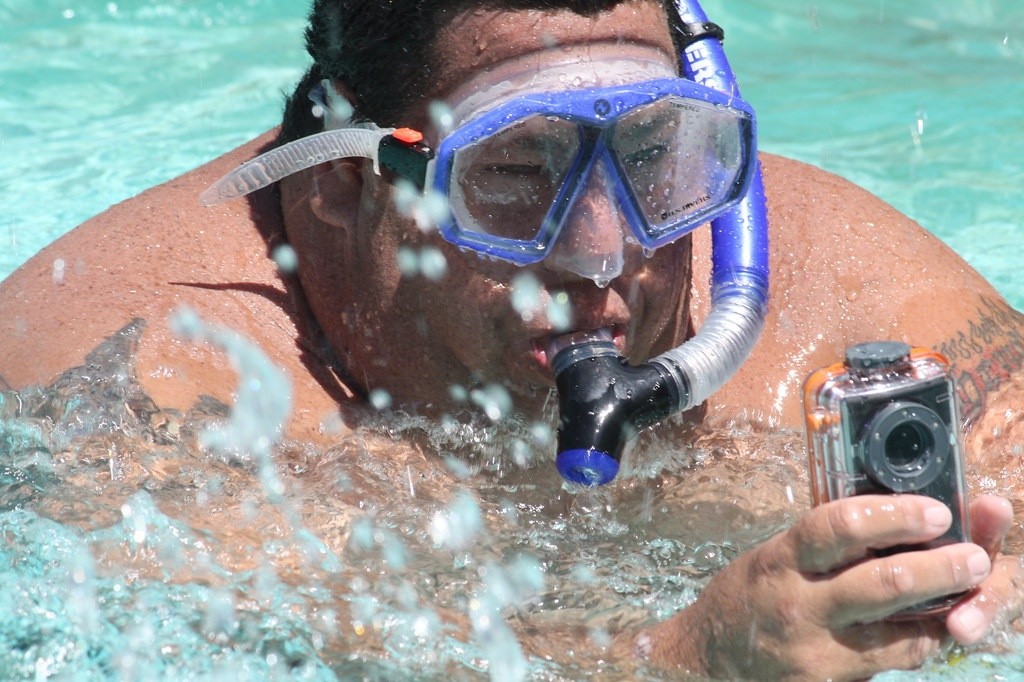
[0,1,1024,682]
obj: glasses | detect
[194,52,759,288]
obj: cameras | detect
[819,359,973,612]
